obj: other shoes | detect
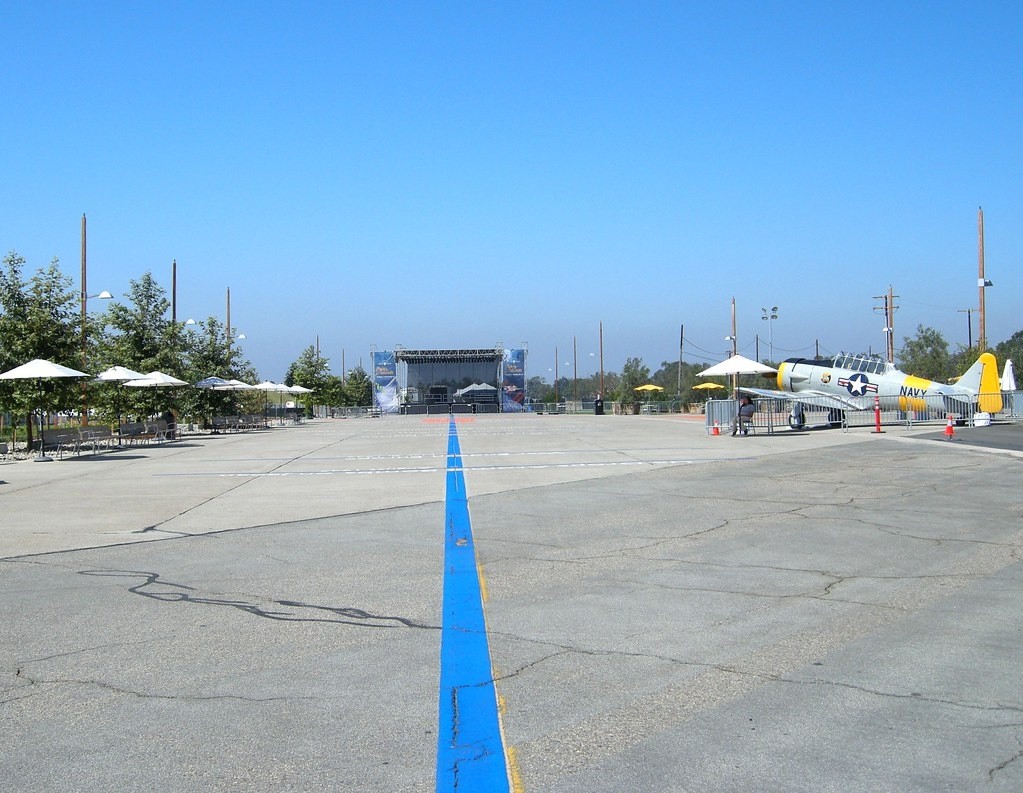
[744,429,748,435]
[731,429,738,436]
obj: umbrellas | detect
[696,353,780,436]
[948,376,962,382]
[122,371,189,444]
[1000,358,1017,418]
[89,363,151,449]
[456,382,498,396]
[192,376,314,434]
[0,356,92,463]
[634,383,667,416]
[693,382,723,407]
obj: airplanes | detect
[762,352,1003,429]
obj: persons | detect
[162,402,179,440]
[731,395,756,436]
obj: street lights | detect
[883,327,894,363]
[761,306,779,362]
[565,362,577,402]
[227,334,247,351]
[342,372,352,386]
[978,277,993,355]
[548,368,558,403]
[172,319,196,429]
[958,308,979,349]
[589,353,604,401]
[80,291,114,427]
[725,335,737,400]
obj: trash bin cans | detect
[595,399,603,415]
[161,411,175,438]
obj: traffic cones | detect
[710,420,721,435]
[942,416,957,436]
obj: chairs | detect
[40,416,273,460]
[735,413,756,435]
